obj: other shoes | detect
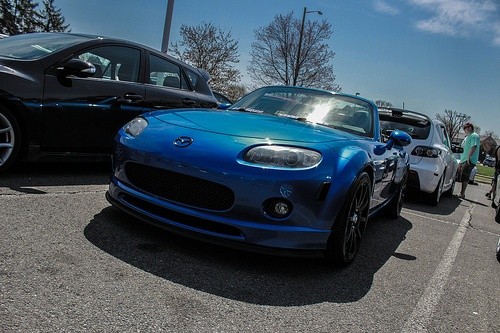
[453,193,465,198]
[485,193,491,199]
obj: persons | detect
[453,122,481,199]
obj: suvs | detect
[0,32,233,177]
[377,106,464,206]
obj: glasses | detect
[464,127,469,129]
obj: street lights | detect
[292,6,324,88]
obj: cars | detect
[483,156,496,168]
[496,232,500,262]
[211,90,232,107]
[491,145,500,223]
[452,144,478,184]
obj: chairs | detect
[334,111,369,137]
[92,64,102,78]
[163,76,179,88]
[118,65,130,81]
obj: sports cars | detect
[104,85,412,268]
